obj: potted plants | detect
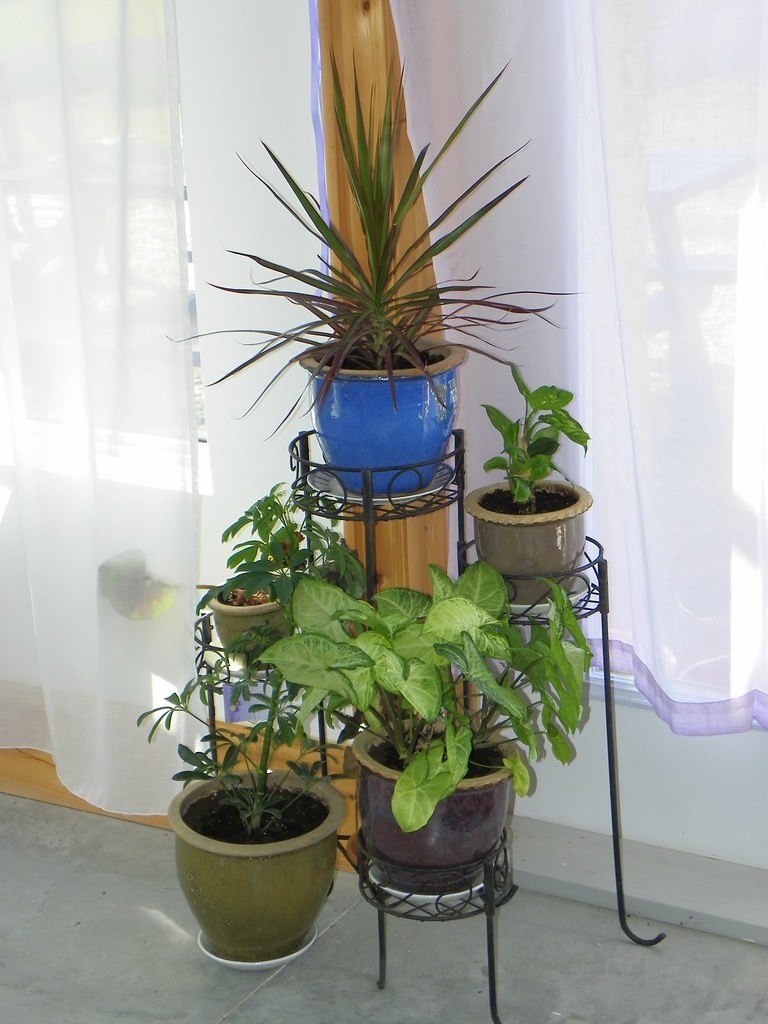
[136,36,598,970]
